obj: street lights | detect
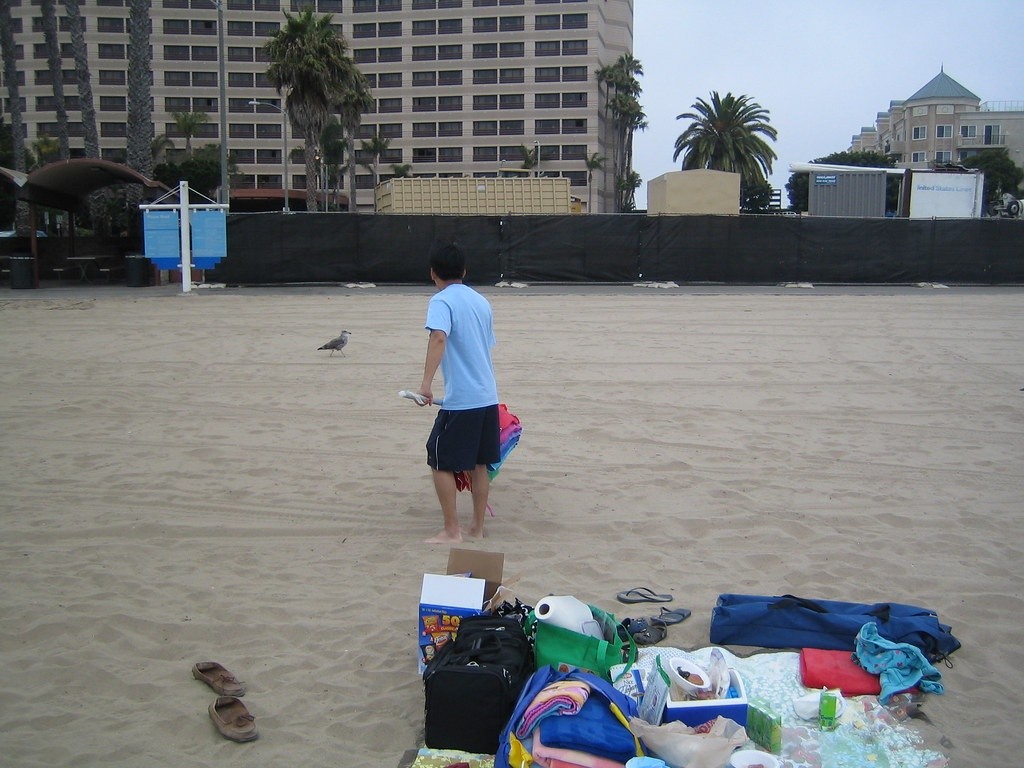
[533,140,540,178]
[248,101,289,214]
[315,152,324,211]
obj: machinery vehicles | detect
[498,168,581,214]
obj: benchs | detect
[52,267,71,281]
[100,266,125,284]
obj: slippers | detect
[617,587,673,602]
[659,606,691,624]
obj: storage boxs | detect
[418,547,506,682]
[663,666,748,723]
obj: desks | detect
[67,255,115,283]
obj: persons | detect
[417,245,500,544]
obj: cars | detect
[0,230,48,237]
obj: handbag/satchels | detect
[523,603,638,683]
[709,594,961,668]
[423,616,535,755]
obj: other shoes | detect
[208,696,258,742]
[192,661,244,697]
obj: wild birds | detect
[317,330,351,357]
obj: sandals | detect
[633,621,667,644]
[617,617,648,642]
